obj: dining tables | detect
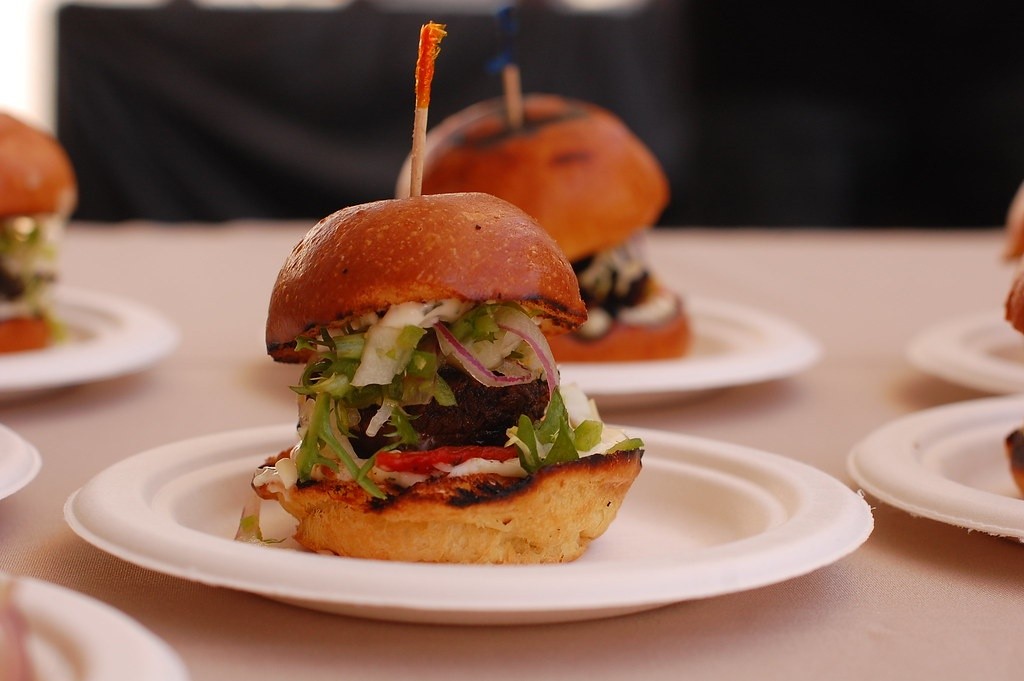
[0,211,1024,681]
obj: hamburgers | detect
[234,92,693,568]
[0,106,80,354]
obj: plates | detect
[846,391,1024,548]
[548,296,821,412]
[67,411,875,622]
[0,565,194,681]
[1,281,179,397]
[903,312,1024,392]
[1,420,41,503]
